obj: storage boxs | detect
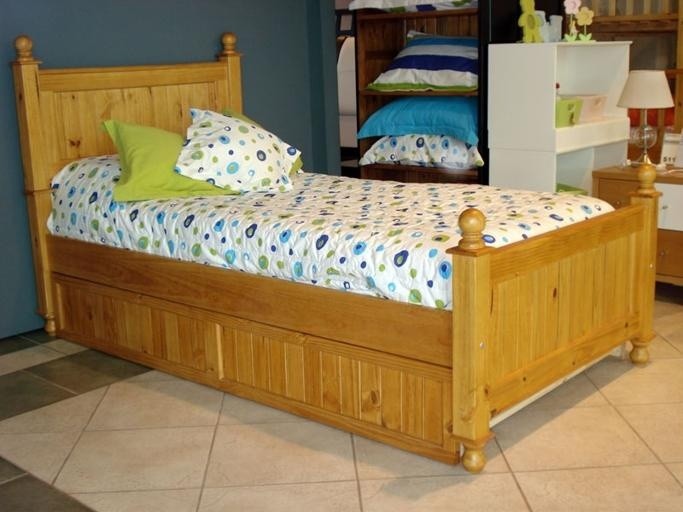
[579,93,606,121]
[556,99,583,126]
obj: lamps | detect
[618,70,675,165]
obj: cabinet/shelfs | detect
[487,40,632,193]
[594,165,683,288]
[355,6,480,178]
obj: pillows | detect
[104,119,239,200]
[175,107,300,194]
[222,106,304,180]
[359,97,480,145]
[359,134,483,174]
[366,31,480,92]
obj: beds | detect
[12,31,663,471]
